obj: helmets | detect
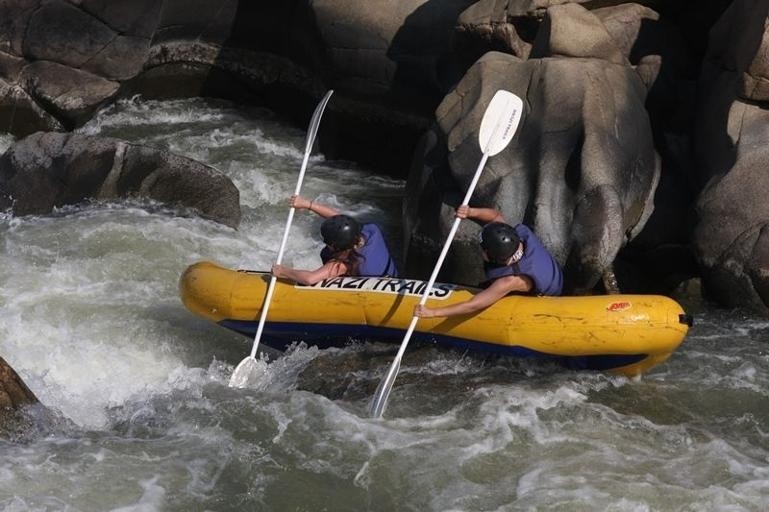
[478,219,520,263]
[316,214,364,252]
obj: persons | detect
[412,203,571,321]
[269,193,399,287]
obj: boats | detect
[178,261,692,379]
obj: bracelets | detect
[307,201,313,210]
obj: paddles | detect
[228,90,334,386]
[368,89,524,421]
